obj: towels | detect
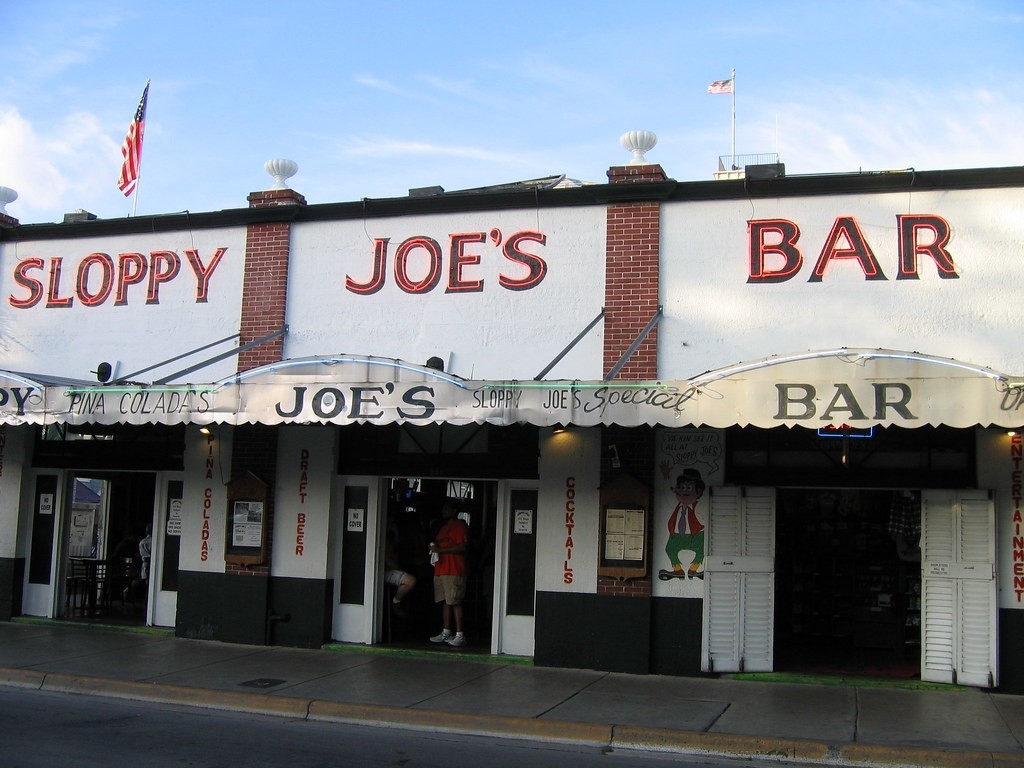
[428,541,439,568]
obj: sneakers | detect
[448,636,468,647]
[430,633,454,643]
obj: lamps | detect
[198,424,213,435]
[552,425,565,435]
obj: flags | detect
[708,79,733,94]
[117,84,149,197]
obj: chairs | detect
[64,554,114,617]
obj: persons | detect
[429,499,468,646]
[112,523,153,603]
[385,513,416,618]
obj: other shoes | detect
[392,603,406,616]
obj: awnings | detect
[0,348,1024,429]
